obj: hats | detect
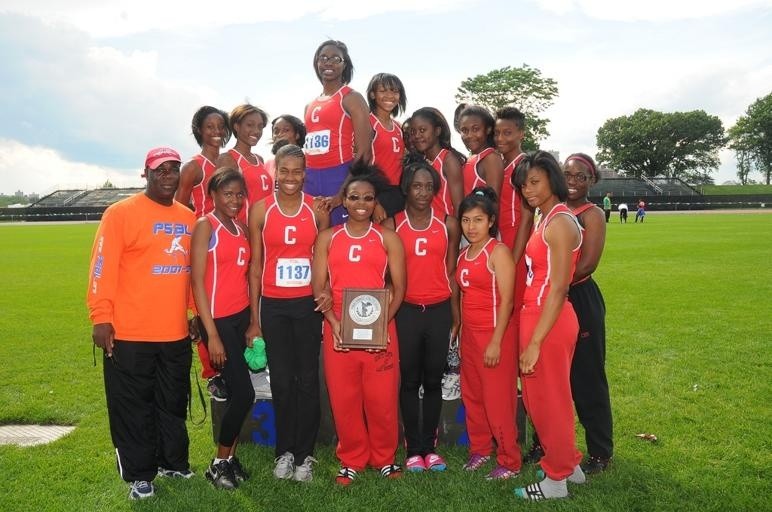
[145,147,182,170]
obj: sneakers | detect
[249,369,272,397]
[206,374,228,402]
[418,384,424,399]
[441,365,462,401]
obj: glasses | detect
[317,54,344,64]
[347,195,373,201]
[563,173,587,182]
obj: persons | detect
[366,72,408,225]
[617,202,628,223]
[635,200,646,223]
[303,40,372,229]
[83,147,198,501]
[170,105,613,500]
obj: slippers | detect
[526,481,571,500]
